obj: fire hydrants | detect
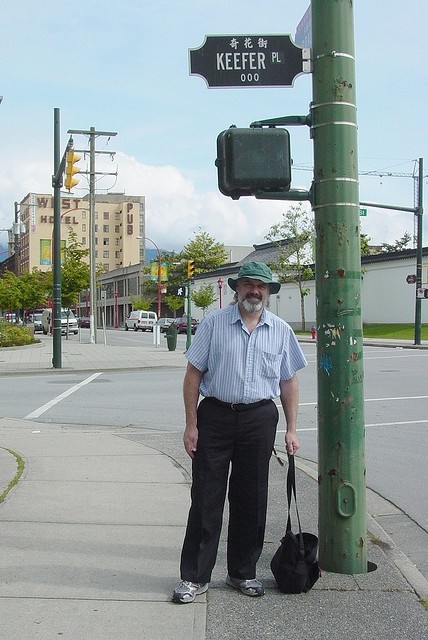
[310,326,317,340]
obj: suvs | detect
[124,310,157,332]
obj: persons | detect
[173,261,307,602]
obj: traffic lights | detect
[216,126,291,199]
[65,148,81,190]
[188,260,195,278]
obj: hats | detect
[227,262,280,294]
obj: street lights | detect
[136,236,161,320]
[217,277,224,310]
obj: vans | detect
[42,308,78,335]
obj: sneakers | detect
[172,580,208,603]
[225,574,263,597]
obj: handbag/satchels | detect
[269,455,320,594]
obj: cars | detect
[27,314,43,334]
[77,317,90,328]
[158,318,179,333]
[5,314,23,324]
[174,318,198,335]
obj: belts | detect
[209,397,269,411]
